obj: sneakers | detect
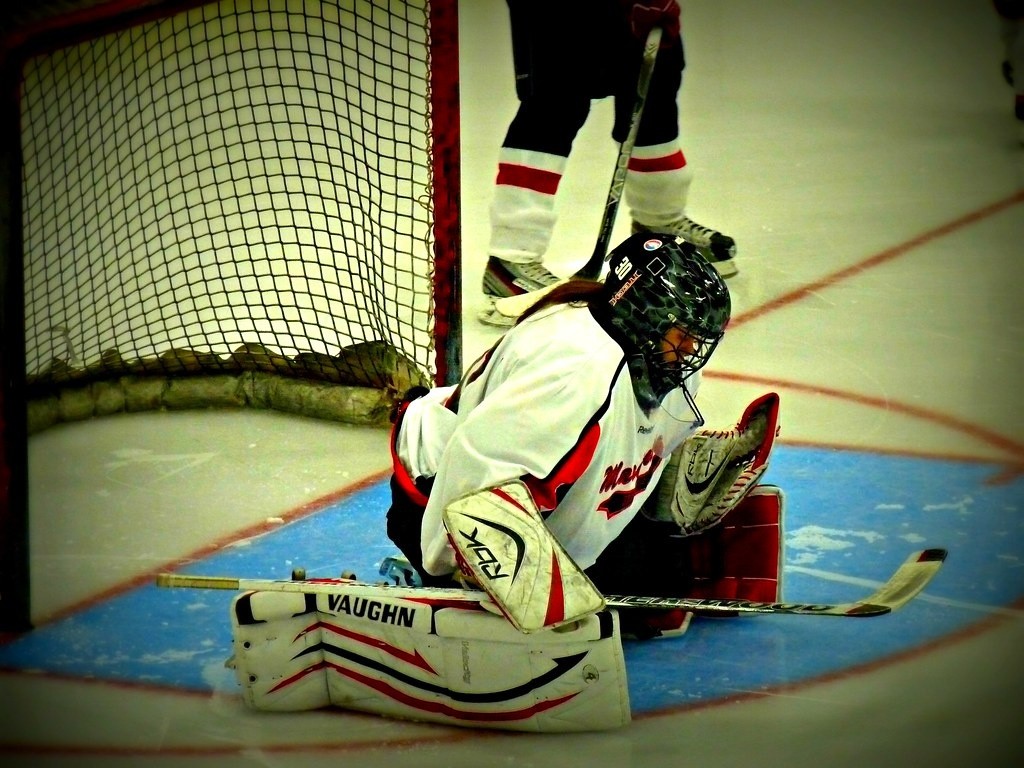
[474,253,562,326]
[629,216,739,279]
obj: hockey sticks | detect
[493,24,665,319]
[155,548,951,619]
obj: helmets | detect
[593,232,730,411]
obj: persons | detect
[476,0,736,327]
[223,234,781,733]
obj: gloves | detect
[632,0,682,48]
